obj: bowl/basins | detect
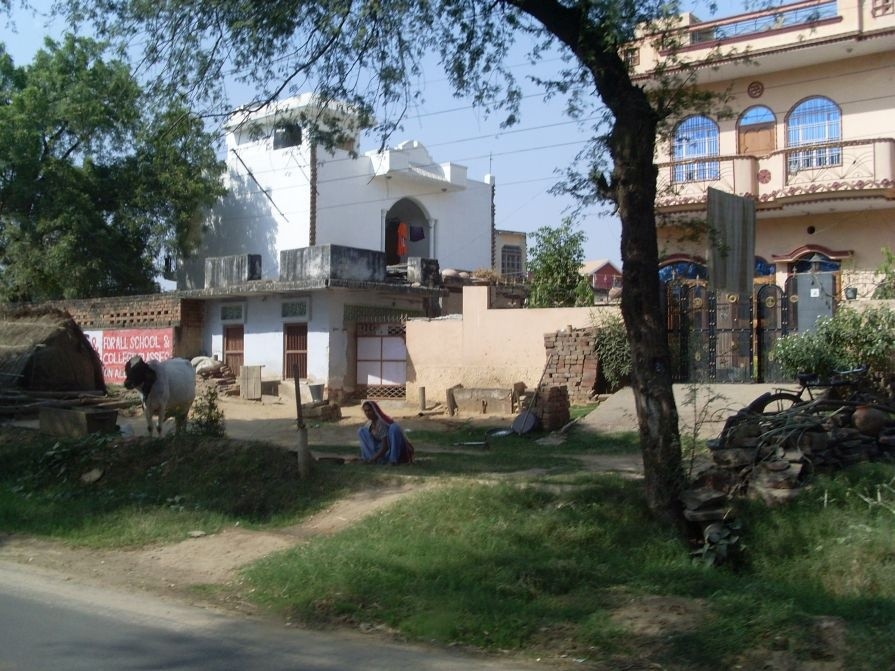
[485,427,514,439]
[513,412,538,435]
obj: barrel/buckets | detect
[309,384,324,403]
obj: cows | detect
[124,356,198,438]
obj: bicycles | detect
[754,364,891,416]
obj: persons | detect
[357,401,410,466]
